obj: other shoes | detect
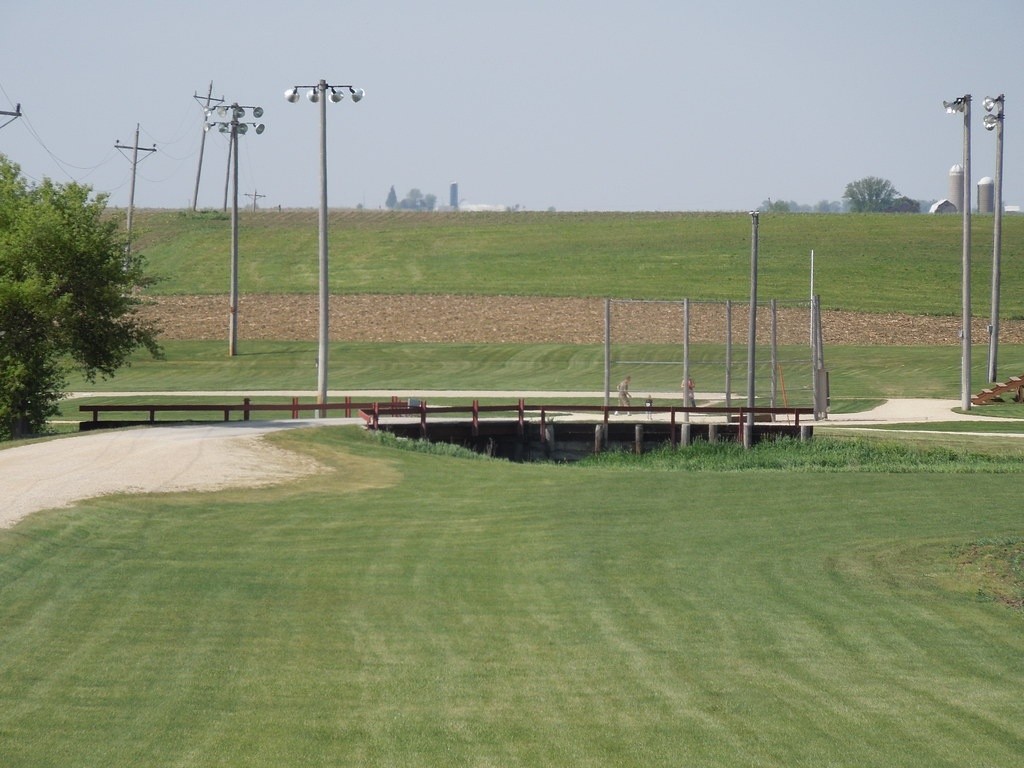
[627,412,631,416]
[614,411,618,416]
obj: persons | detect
[681,379,696,407]
[645,395,653,420]
[613,376,632,416]
